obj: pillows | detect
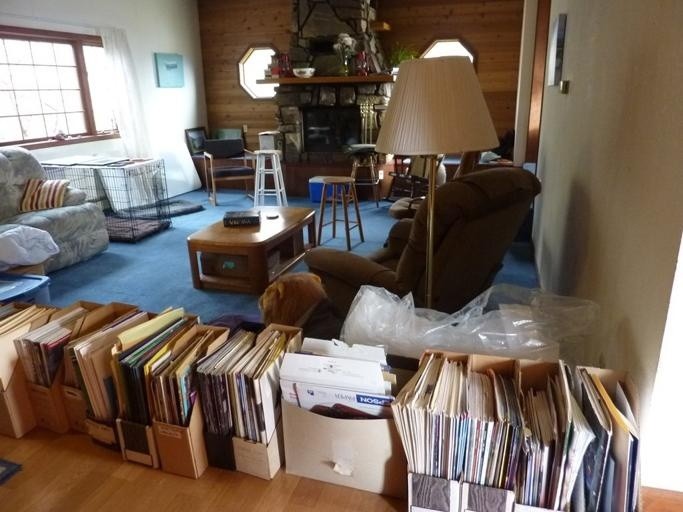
[17,177,69,213]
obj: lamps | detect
[373,55,500,311]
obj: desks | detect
[443,153,512,178]
[191,151,281,199]
[0,417,683,512]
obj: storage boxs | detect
[0,272,53,307]
[309,175,349,203]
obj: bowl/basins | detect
[292,67,315,79]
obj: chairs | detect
[201,137,258,207]
[381,153,444,204]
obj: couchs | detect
[305,166,540,320]
[0,145,108,275]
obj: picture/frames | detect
[154,52,185,88]
[184,125,208,156]
[544,13,567,87]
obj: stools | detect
[346,143,380,208]
[317,177,364,250]
[253,148,288,207]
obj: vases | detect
[337,54,351,76]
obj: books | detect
[390,353,639,512]
[222,210,260,227]
[0,299,396,446]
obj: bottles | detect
[262,52,290,80]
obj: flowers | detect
[332,31,356,72]
